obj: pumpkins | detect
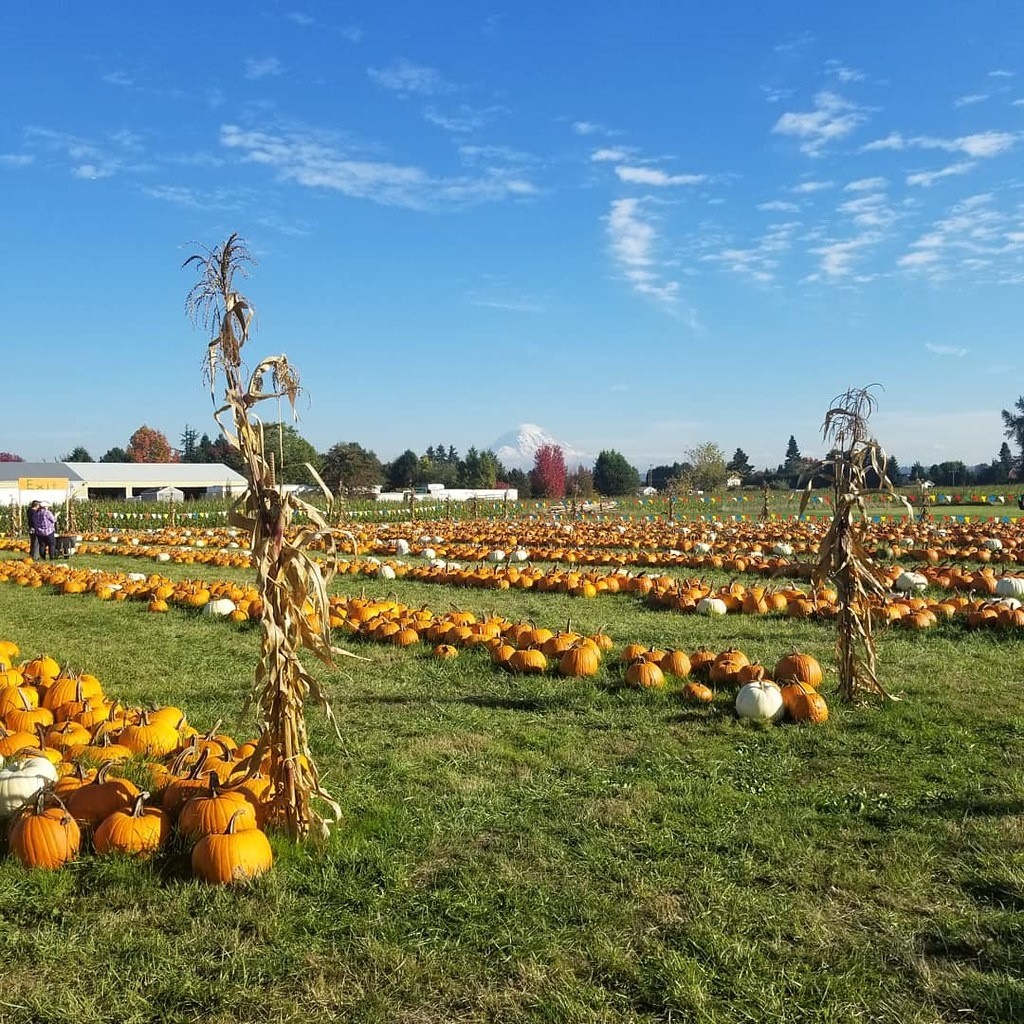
[0,557,828,723]
[0,640,313,881]
[0,518,1024,629]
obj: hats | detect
[40,500,53,508]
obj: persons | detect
[36,502,57,560]
[27,500,41,561]
